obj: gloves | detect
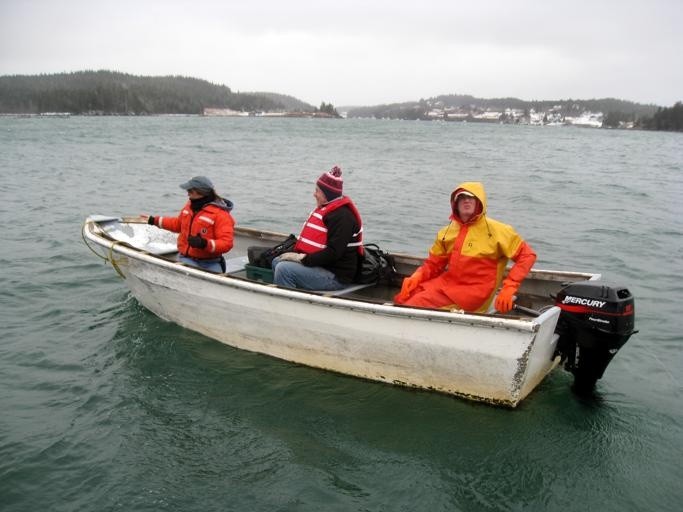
[400,271,422,299]
[494,279,521,313]
[277,252,307,264]
[188,232,208,250]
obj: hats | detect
[179,176,215,193]
[454,188,476,202]
[316,166,344,195]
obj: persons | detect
[136,175,234,276]
[271,165,366,291]
[390,177,536,320]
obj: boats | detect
[81,212,635,411]
